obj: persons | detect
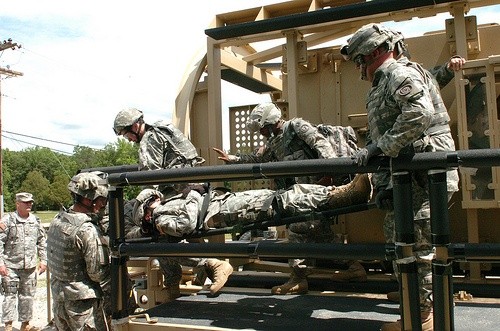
[212,100,367,295]
[427,54,466,91]
[339,21,439,331]
[113,107,233,308]
[85,171,145,331]
[0,192,49,331]
[46,172,112,331]
[131,168,375,236]
[382,27,460,304]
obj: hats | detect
[16,192,35,202]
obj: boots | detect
[271,268,308,294]
[388,290,401,303]
[161,283,181,305]
[4,321,13,331]
[205,258,233,294]
[328,174,370,211]
[380,306,435,331]
[332,260,367,280]
[332,173,361,191]
[20,321,39,331]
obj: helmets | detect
[246,102,282,131]
[113,108,143,138]
[67,172,109,201]
[342,23,394,81]
[388,27,406,59]
[132,188,163,226]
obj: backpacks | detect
[288,116,361,186]
